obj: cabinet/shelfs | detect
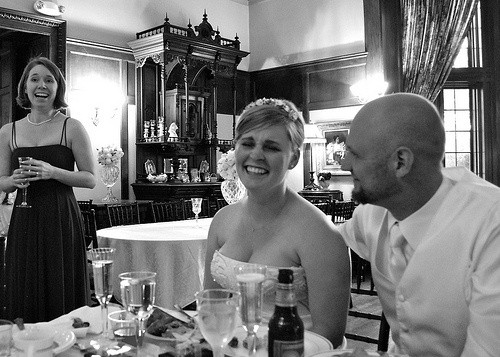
[126,8,250,221]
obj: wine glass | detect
[197,289,241,357]
[16,157,33,210]
[233,263,267,356]
[191,198,202,228]
[91,247,116,341]
[119,271,157,357]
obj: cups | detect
[107,312,144,352]
[0,320,13,357]
[12,329,57,356]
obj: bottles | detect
[268,269,304,357]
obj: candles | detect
[310,147,313,172]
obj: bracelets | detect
[27,110,60,125]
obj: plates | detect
[142,329,196,343]
[20,319,334,356]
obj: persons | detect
[337,93,500,357]
[204,97,351,349]
[0,57,95,325]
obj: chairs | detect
[76,197,391,353]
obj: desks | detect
[88,217,214,310]
[0,304,333,357]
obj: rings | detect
[36,172,38,176]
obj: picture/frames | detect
[316,120,352,175]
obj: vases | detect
[100,164,120,203]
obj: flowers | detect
[97,146,124,180]
